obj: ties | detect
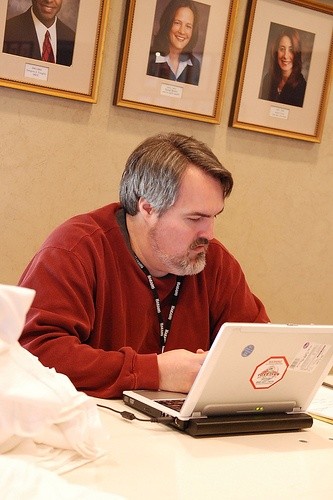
[41,30,55,63]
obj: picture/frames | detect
[0,0,111,108]
[227,1,333,144]
[114,0,242,129]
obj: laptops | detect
[122,321,333,437]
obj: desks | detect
[5,358,333,463]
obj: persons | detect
[146,0,201,87]
[13,130,271,400]
[260,27,307,109]
[2,0,77,68]
